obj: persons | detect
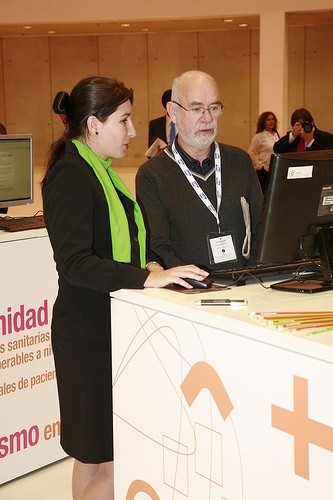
[134,71,264,276]
[41,77,209,500]
[147,90,333,196]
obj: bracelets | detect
[145,262,159,268]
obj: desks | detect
[109,267,333,500]
[0,227,71,485]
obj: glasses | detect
[172,100,225,118]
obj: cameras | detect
[300,121,312,132]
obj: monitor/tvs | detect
[0,134,34,214]
[256,150,333,293]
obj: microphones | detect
[272,128,280,139]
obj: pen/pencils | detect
[247,311,333,337]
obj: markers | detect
[195,298,247,306]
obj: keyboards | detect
[0,215,46,231]
[209,258,321,287]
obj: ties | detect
[296,138,305,152]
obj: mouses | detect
[173,278,211,288]
